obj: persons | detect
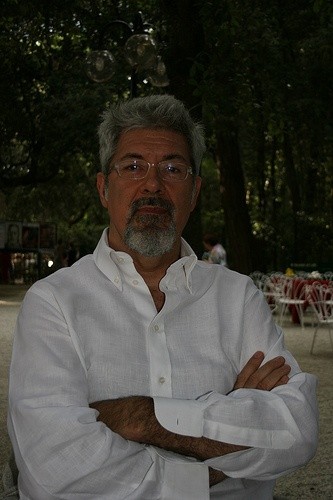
[11,93,317,499]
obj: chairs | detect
[249,271,333,356]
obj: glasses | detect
[105,158,194,182]
